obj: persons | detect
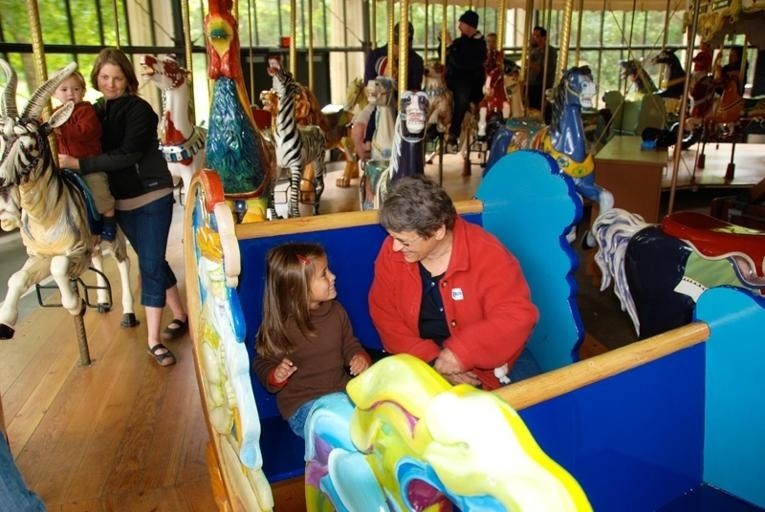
[364,177,538,393]
[251,240,369,437]
[720,44,747,96]
[44,67,119,243]
[353,9,557,161]
[54,47,190,365]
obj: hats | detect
[393,21,414,42]
[459,11,479,28]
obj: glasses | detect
[395,236,423,248]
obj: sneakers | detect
[161,315,187,340]
[147,343,176,367]
[446,134,457,145]
[101,216,117,240]
